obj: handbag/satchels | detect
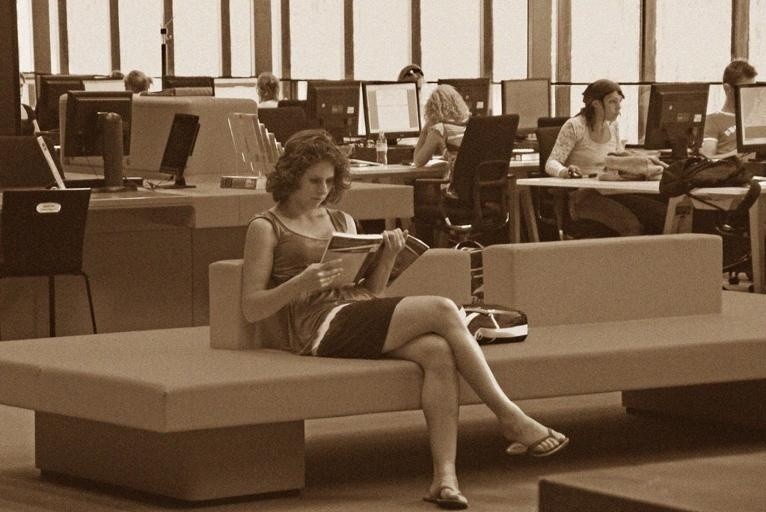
[457,304,528,343]
[660,155,752,196]
[601,150,663,180]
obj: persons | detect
[544,78,668,237]
[20,72,35,120]
[411,85,472,247]
[124,70,155,94]
[698,59,760,163]
[255,71,287,110]
[241,130,570,508]
[395,64,428,87]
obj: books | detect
[319,232,430,288]
[410,159,448,169]
[219,175,268,190]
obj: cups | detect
[703,138,719,157]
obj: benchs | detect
[0,232,766,500]
[537,454,765,511]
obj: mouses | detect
[569,170,582,178]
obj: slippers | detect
[507,427,569,457]
[423,486,467,509]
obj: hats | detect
[583,80,622,107]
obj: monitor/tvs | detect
[47,90,143,189]
[500,78,551,136]
[22,71,309,131]
[643,83,709,164]
[614,83,653,148]
[550,82,590,118]
[362,80,421,145]
[437,78,491,117]
[734,82,766,161]
[159,112,200,188]
[307,81,361,146]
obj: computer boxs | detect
[739,160,766,178]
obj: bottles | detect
[101,111,125,193]
[376,130,387,164]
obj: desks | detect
[0,105,766,317]
